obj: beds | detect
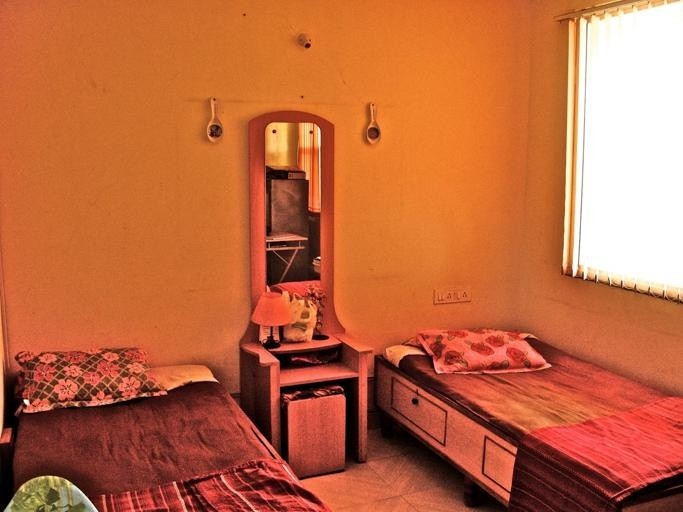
[11,365,331,512]
[375,333,683,511]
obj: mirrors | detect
[238,111,346,340]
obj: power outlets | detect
[434,288,472,306]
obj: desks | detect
[239,332,375,464]
[266,232,308,282]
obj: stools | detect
[282,386,348,480]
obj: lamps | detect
[250,291,293,348]
[295,32,312,49]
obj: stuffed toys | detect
[276,294,321,342]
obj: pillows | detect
[15,347,168,413]
[417,328,552,374]
